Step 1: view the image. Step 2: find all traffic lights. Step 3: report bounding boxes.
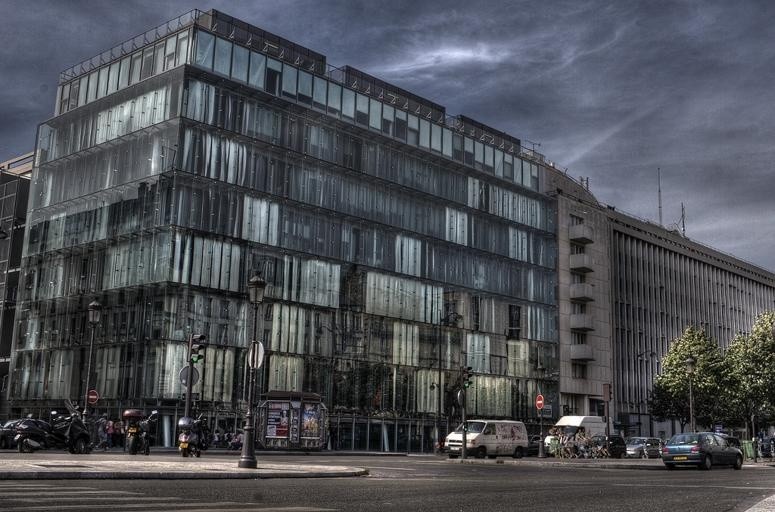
[460,366,473,389]
[190,334,207,362]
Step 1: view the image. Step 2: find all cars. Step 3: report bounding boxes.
[626,437,663,458]
[662,432,744,469]
[593,434,627,460]
[529,434,540,450]
[2,418,68,446]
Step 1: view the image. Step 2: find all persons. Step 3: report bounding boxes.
[281,408,317,443]
[545,426,611,460]
[87,412,128,452]
[216,425,244,451]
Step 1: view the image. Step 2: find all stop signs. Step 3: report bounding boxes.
[88,389,99,404]
[536,395,544,410]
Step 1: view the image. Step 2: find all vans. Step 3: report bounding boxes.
[444,420,528,458]
[544,415,605,454]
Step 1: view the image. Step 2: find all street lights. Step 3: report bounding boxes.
[83,301,102,438]
[685,355,695,434]
[536,363,547,458]
[238,277,267,467]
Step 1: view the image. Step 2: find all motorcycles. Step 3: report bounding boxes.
[123,409,156,455]
[177,413,203,458]
[16,418,49,453]
[65,413,93,453]
